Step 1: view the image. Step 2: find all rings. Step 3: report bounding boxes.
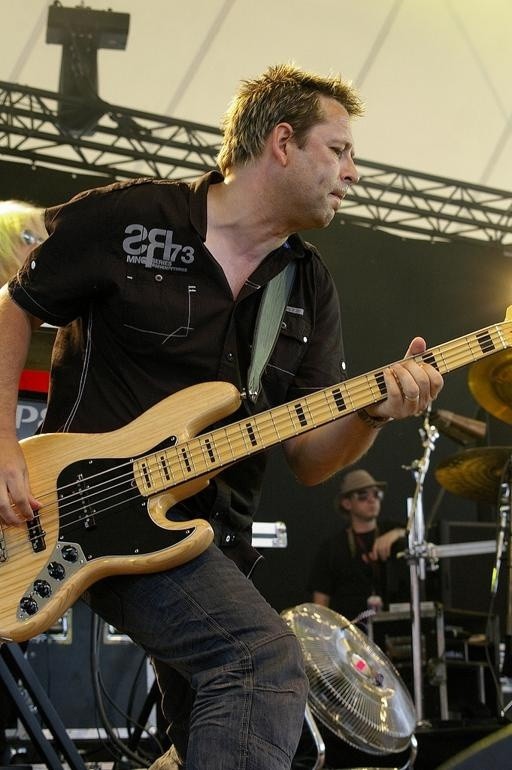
[404,396,419,402]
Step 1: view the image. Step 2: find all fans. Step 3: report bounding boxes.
[279,606,422,768]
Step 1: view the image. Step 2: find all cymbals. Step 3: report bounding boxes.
[468,346,511,424]
[436,447,511,507]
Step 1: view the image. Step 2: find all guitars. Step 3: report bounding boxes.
[1,319,512,643]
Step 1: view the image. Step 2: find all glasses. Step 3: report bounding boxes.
[18,228,45,248]
[353,490,385,501]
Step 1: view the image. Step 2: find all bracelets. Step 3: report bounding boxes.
[355,410,394,430]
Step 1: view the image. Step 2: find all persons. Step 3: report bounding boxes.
[0,66,445,770]
[0,200,49,288]
[309,470,409,652]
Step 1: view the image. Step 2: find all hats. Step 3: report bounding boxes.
[337,469,389,495]
